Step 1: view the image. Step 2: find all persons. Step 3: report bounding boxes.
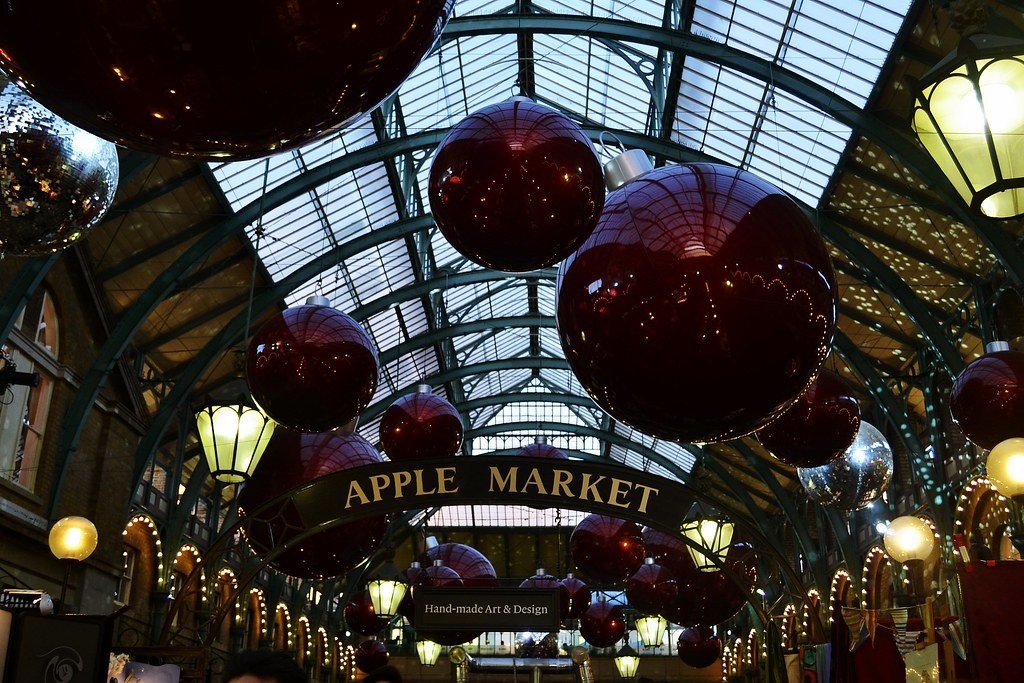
[219,648,309,683]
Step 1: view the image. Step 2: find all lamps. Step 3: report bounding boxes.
[634,612,668,650]
[188,155,278,485]
[614,594,642,681]
[905,0,1024,219]
[0,517,130,623]
[366,324,409,621]
[415,508,443,668]
[883,515,934,605]
[678,446,735,575]
[987,437,1024,560]
